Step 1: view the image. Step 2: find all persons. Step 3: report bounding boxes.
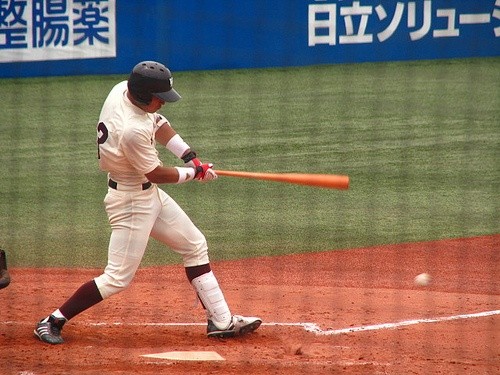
[33,60,262,344]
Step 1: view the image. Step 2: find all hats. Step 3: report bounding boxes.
[128,60,182,105]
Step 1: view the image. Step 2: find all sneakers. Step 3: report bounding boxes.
[33,317,65,345]
[207,314,262,339]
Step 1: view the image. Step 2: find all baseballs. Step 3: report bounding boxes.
[413,273,431,287]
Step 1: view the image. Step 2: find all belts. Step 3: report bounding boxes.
[108,178,152,191]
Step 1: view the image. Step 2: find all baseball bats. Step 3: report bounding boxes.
[213,170,350,189]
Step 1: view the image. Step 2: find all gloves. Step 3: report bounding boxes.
[181,151,203,168]
[192,163,218,184]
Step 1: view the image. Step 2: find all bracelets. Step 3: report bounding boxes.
[166,134,190,159]
[175,166,195,184]
